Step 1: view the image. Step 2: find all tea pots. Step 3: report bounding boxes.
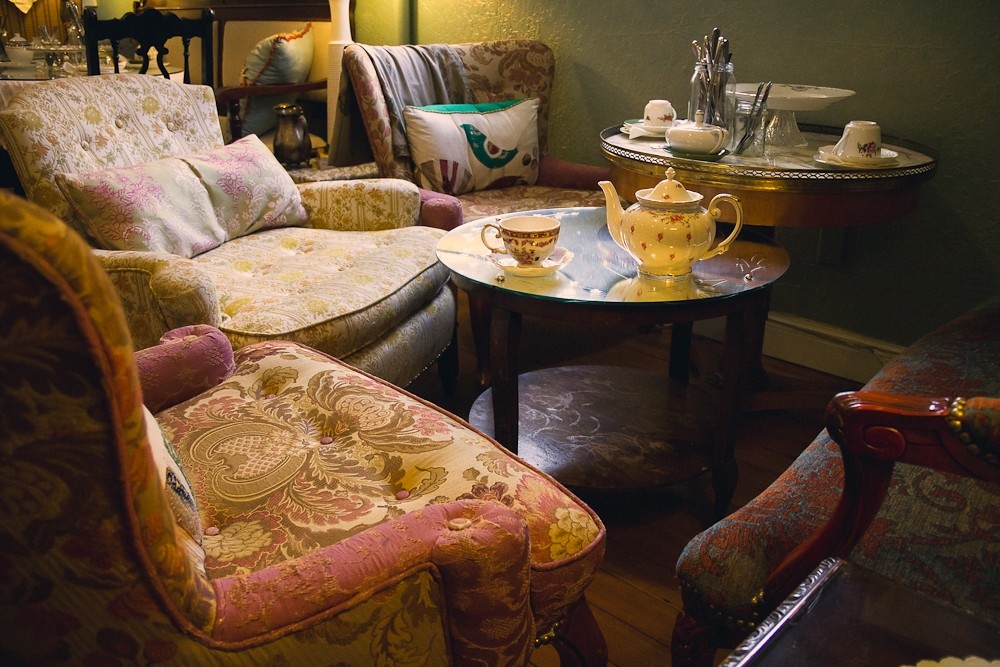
[597,167,744,278]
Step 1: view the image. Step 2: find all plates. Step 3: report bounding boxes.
[76,67,126,73]
[486,246,574,277]
[130,63,170,69]
[812,144,900,168]
[0,62,37,67]
[621,117,666,139]
[724,81,857,111]
[667,148,730,162]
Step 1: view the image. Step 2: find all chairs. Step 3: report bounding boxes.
[214,9,332,171]
[84,8,214,87]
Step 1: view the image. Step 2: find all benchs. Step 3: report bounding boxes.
[668,296,1000,667]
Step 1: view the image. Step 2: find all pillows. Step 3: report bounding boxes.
[402,98,540,196]
[239,21,314,137]
[53,134,307,258]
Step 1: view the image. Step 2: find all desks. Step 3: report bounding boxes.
[0,59,185,84]
[720,555,1000,666]
[597,121,942,414]
[433,207,790,529]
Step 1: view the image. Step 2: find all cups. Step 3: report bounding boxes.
[481,215,560,266]
[643,99,676,127]
[837,121,882,158]
[664,109,730,154]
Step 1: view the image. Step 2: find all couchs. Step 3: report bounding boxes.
[343,40,612,223]
[0,190,609,667]
[0,73,462,404]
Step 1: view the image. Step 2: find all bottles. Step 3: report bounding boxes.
[689,61,736,149]
[736,97,767,158]
